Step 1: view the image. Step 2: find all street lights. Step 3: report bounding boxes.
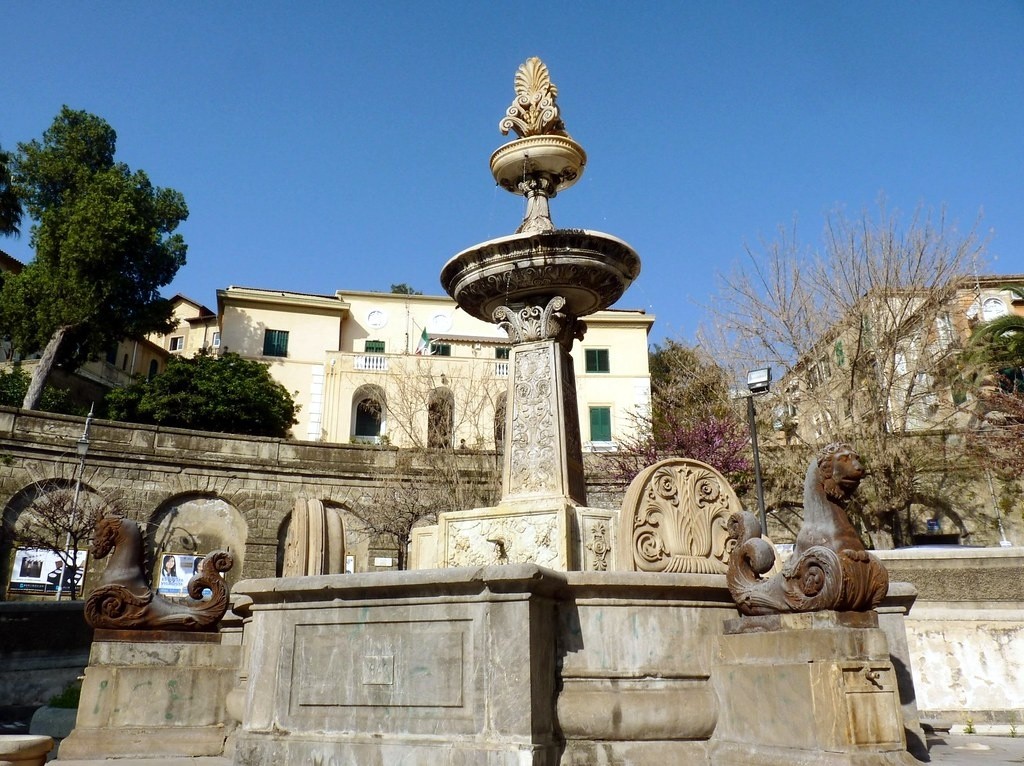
[733,369,771,543]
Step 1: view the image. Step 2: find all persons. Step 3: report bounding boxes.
[193,557,205,576]
[162,556,177,577]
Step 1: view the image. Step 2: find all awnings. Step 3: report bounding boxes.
[425,333,512,344]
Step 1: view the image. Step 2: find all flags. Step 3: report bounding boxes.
[415,326,429,354]
